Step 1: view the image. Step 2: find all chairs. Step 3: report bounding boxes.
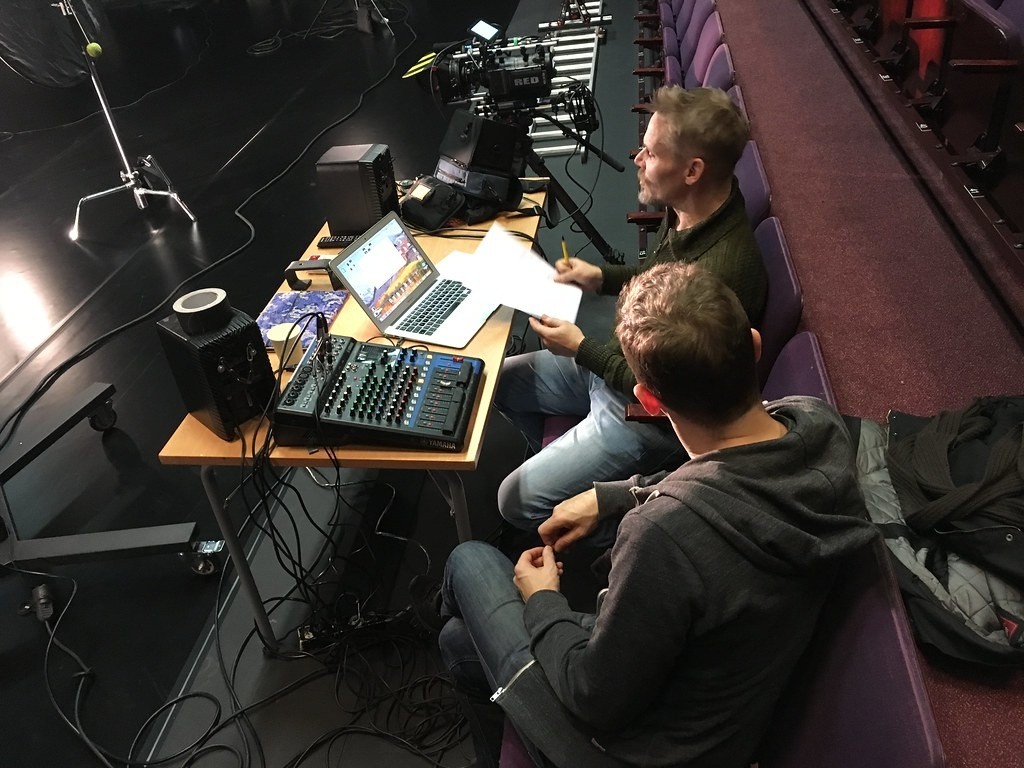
[630,0,949,768]
[907,0,1024,167]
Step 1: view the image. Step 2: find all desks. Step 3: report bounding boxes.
[157,178,549,654]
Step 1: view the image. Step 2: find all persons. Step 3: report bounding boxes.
[406,261,881,768]
[491,86,769,533]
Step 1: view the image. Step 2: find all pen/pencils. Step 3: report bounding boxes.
[561,234,572,269]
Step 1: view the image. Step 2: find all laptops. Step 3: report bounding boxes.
[328,210,502,349]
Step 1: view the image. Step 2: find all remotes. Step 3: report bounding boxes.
[319,235,363,248]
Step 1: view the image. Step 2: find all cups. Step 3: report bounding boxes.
[267,322,303,368]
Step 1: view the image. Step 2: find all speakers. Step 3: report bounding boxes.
[316,143,401,237]
[156,302,280,443]
[438,108,520,177]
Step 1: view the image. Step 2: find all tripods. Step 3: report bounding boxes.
[303,1,395,41]
[51,0,198,236]
